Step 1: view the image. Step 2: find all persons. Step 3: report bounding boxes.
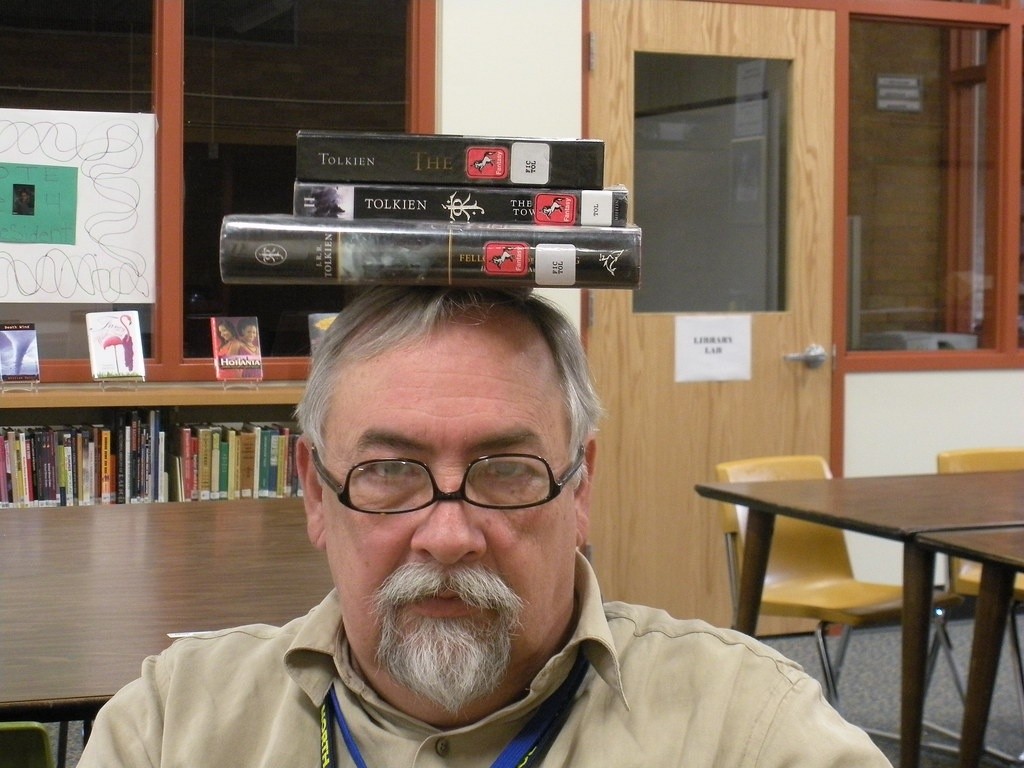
[217,320,260,377]
[78,285,896,768]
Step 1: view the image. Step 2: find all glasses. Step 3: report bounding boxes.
[310,441,585,515]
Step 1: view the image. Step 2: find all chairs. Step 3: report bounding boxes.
[0,721,68,768]
[923,446,1024,768]
[712,456,969,756]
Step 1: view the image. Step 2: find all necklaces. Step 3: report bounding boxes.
[247,343,255,347]
[244,341,257,352]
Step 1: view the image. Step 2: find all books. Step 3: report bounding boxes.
[0,323,40,380]
[309,314,340,356]
[219,131,643,290]
[0,410,305,509]
[86,311,146,381]
[210,317,263,381]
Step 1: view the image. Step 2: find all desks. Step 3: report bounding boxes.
[0,383,307,407]
[0,497,335,722]
[693,468,1024,768]
